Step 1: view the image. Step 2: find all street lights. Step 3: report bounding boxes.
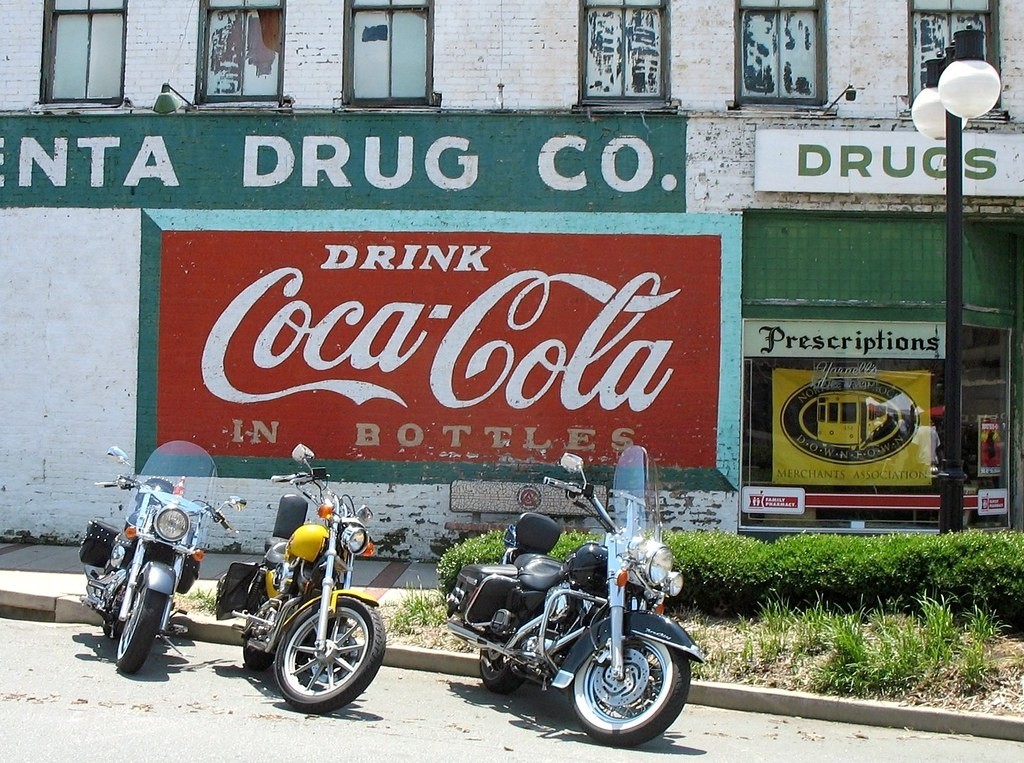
[911,22,1002,535]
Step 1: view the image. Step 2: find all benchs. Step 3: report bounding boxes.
[444,479,608,541]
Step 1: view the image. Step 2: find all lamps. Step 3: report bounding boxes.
[822,83,857,117]
[152,83,194,114]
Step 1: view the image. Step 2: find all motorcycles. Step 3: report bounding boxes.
[213,443,387,715]
[77,439,247,674]
[442,443,706,750]
